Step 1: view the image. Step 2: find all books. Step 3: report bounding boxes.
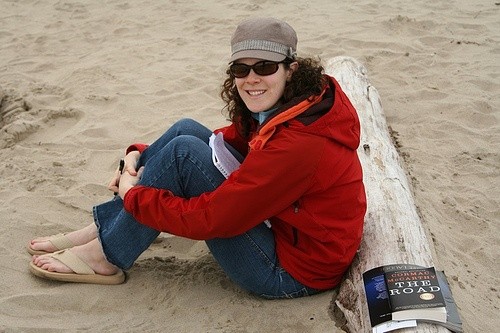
[362,264,463,333]
[209,132,271,228]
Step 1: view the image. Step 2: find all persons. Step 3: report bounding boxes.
[25,17,366,299]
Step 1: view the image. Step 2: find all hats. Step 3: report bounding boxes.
[228,17,298,65]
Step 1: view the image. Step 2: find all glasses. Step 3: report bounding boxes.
[230,59,291,78]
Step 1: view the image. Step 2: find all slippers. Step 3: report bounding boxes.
[27,232,77,257]
[28,246,124,285]
